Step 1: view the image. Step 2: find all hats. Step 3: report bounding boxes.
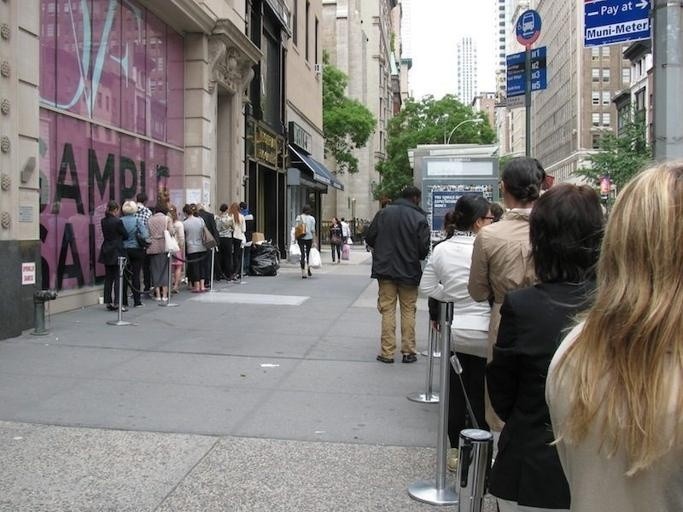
[301,204,313,213]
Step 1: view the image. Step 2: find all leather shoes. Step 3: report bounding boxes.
[106,287,208,312]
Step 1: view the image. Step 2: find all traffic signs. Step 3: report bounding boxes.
[504,44,547,97]
[515,9,543,47]
[583,0,652,48]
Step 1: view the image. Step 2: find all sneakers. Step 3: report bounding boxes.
[403,354,417,363]
[377,355,394,363]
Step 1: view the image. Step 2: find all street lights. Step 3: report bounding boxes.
[444,114,484,142]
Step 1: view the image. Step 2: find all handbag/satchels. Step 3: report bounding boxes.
[308,242,321,269]
[199,216,217,249]
[163,216,181,253]
[134,219,152,247]
[295,215,306,240]
[347,236,353,244]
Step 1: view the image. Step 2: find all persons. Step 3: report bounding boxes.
[362,220,371,252]
[295,204,317,279]
[365,187,430,364]
[416,157,683,511]
[96,190,254,312]
[329,217,344,264]
[340,217,351,242]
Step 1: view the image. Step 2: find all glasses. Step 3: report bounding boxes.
[473,213,495,223]
